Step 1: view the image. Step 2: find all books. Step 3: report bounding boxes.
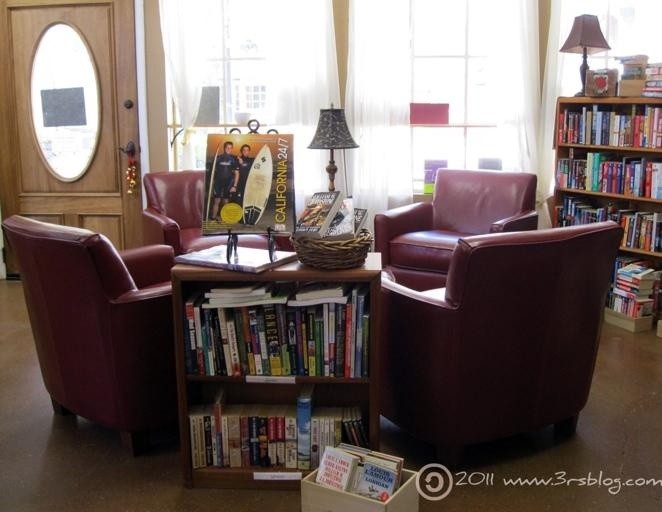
[294,192,368,243]
[554,56,662,327]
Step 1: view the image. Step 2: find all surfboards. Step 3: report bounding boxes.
[242,144,273,226]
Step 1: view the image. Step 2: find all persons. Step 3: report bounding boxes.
[230,144,255,204]
[210,141,239,223]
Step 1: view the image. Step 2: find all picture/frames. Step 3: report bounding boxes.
[585,68,618,97]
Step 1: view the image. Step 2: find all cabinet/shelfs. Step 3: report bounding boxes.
[550,97,662,339]
[169,251,382,487]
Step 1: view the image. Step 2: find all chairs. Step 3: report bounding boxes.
[362,217,622,474]
[1,214,197,459]
[372,169,542,291]
[142,171,278,265]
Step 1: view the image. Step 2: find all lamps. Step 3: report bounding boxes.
[559,13,611,97]
[306,103,358,191]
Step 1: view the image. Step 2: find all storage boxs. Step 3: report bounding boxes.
[299,463,420,512]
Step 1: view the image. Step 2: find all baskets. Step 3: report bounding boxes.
[289,229,375,271]
